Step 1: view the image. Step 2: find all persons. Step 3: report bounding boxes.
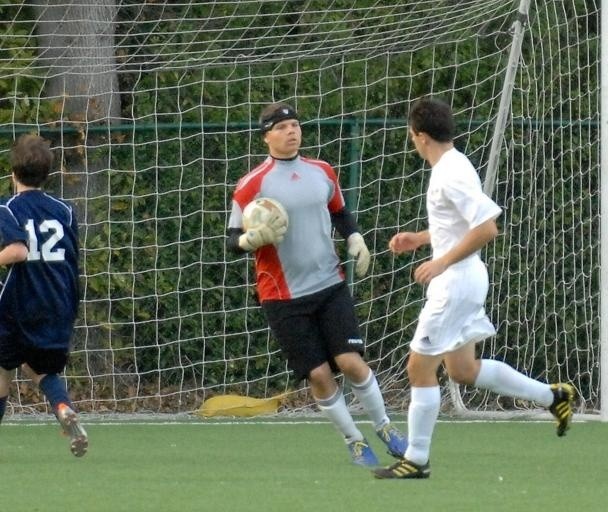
[224,99,409,468]
[371,95,575,480]
[0,129,88,457]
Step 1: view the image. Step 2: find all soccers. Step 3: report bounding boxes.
[241,197,290,236]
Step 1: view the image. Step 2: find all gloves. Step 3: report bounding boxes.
[238,208,287,253]
[346,231,371,278]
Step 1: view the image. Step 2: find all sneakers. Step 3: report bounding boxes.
[373,416,409,460]
[344,431,380,468]
[544,382,575,438]
[58,403,89,458]
[371,457,431,480]
